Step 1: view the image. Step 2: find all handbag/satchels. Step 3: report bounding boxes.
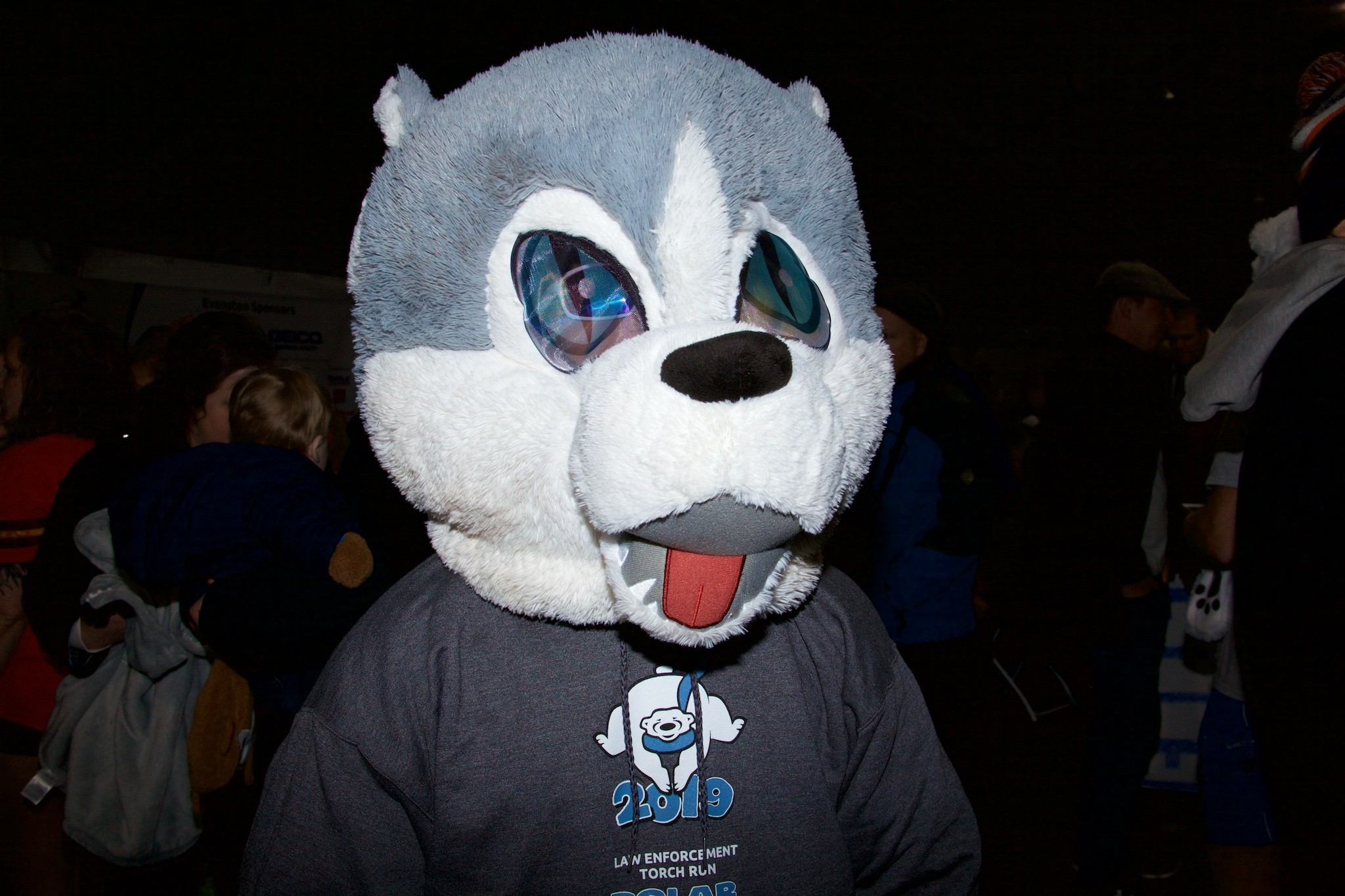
[188,656,260,816]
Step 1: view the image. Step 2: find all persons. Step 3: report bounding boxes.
[1,307,434,896]
[832,285,976,642]
[1019,48,1345,896]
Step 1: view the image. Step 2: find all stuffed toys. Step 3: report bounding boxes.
[236,28,986,896]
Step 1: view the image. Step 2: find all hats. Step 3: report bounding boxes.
[1291,52,1345,149]
[1100,263,1192,302]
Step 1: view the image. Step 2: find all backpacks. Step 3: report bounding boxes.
[876,384,1015,555]
